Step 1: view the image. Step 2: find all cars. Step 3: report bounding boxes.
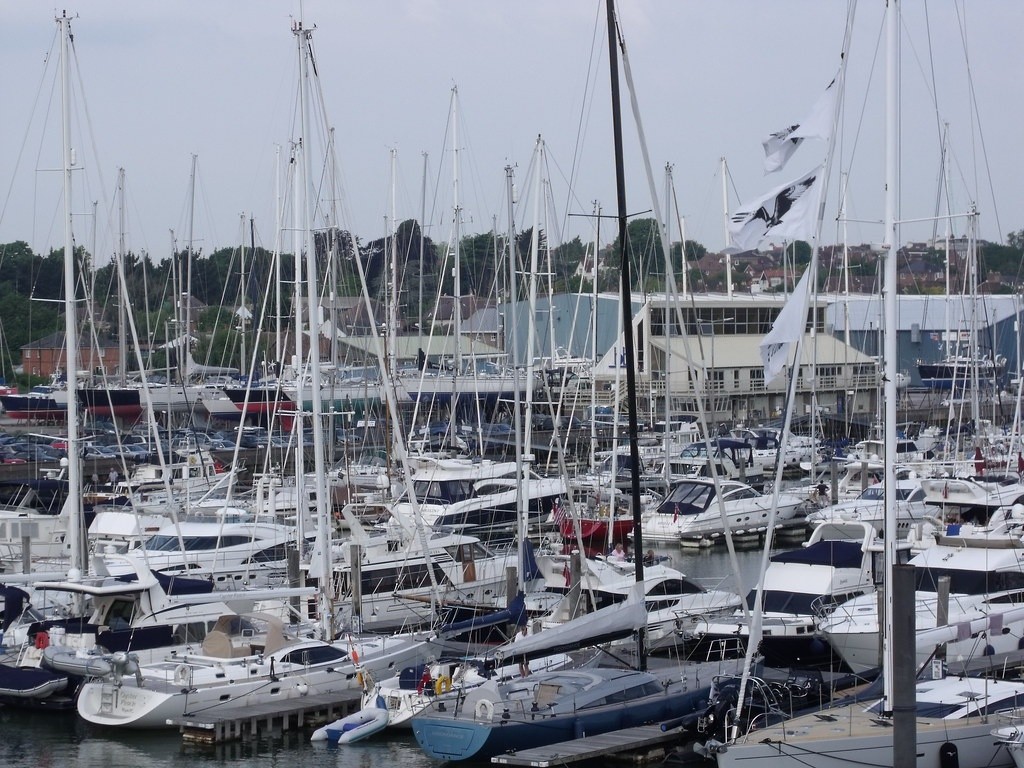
[261,436,285,447]
[482,423,516,437]
[223,432,269,447]
[520,414,548,431]
[559,414,592,429]
[277,435,310,447]
[80,420,123,435]
[80,446,117,461]
[103,443,152,462]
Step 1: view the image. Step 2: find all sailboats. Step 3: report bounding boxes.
[0,2,1024,768]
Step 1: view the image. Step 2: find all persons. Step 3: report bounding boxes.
[612,543,625,558]
[812,480,831,508]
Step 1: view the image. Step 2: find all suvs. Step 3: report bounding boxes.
[589,413,629,429]
[311,428,362,447]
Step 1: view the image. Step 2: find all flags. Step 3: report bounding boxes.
[758,262,811,388]
[762,68,839,176]
[673,507,679,523]
[562,566,571,586]
[729,159,824,251]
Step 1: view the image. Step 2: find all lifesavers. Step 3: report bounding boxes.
[434,674,451,696]
[475,698,495,721]
[34,632,49,649]
[173,664,190,683]
